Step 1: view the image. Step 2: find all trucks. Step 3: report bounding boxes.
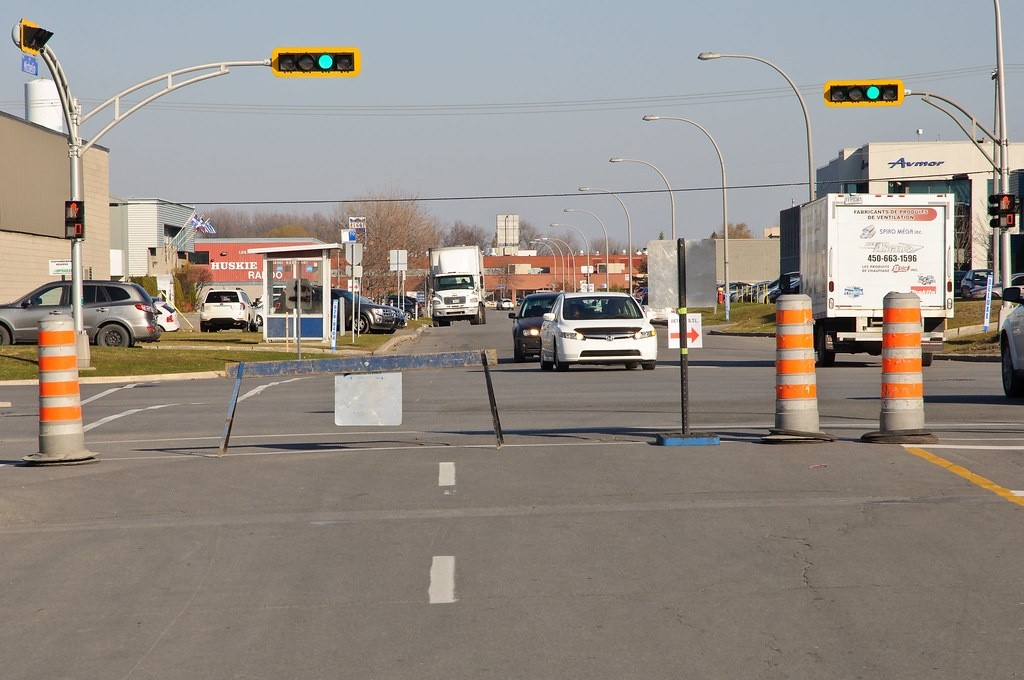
[799,193,955,367]
[428,244,486,327]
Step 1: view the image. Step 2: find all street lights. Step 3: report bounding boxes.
[578,186,634,297]
[528,237,577,293]
[609,157,676,240]
[563,208,609,292]
[641,114,731,323]
[550,223,590,293]
[698,51,815,202]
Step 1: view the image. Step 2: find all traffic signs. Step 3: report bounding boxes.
[668,313,702,350]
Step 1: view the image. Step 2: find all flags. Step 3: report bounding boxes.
[189,214,216,236]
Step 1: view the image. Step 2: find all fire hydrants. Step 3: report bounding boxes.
[718,287,724,304]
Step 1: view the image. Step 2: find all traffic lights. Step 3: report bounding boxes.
[21,17,54,58]
[823,79,905,107]
[65,200,85,239]
[270,46,361,78]
[998,195,1017,228]
[988,194,1001,228]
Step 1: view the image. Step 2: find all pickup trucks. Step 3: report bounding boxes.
[497,298,514,310]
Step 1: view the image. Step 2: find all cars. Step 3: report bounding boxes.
[331,288,407,336]
[999,287,1024,401]
[717,270,800,304]
[153,300,180,333]
[517,299,524,306]
[508,292,597,363]
[254,285,285,327]
[538,291,657,372]
[954,268,1024,302]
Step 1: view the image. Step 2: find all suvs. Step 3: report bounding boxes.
[0,279,161,348]
[373,294,424,321]
[199,287,257,333]
